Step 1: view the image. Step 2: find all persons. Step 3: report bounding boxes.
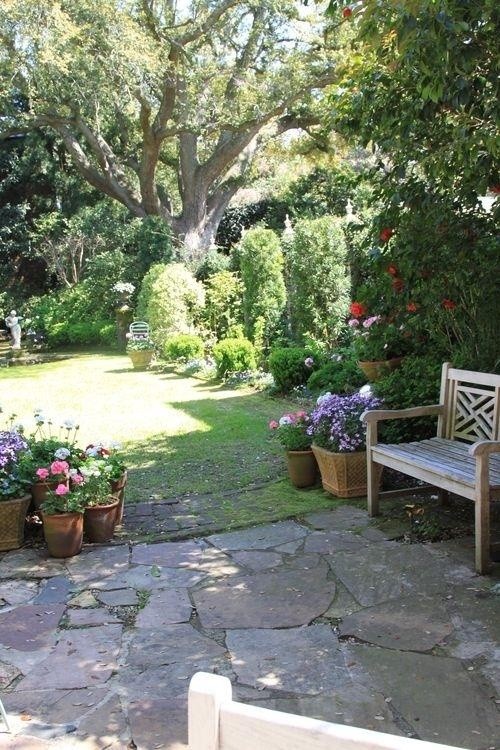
[5,310,23,349]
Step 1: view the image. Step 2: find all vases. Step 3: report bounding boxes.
[310,444,384,499]
[286,451,317,489]
[127,350,155,369]
[0,469,127,558]
[119,291,129,303]
[355,356,406,383]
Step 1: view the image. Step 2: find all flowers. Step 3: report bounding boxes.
[348,314,405,362]
[125,333,154,352]
[0,405,130,514]
[111,282,136,293]
[266,392,384,452]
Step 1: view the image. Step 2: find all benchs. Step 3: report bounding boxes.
[359,361,500,576]
